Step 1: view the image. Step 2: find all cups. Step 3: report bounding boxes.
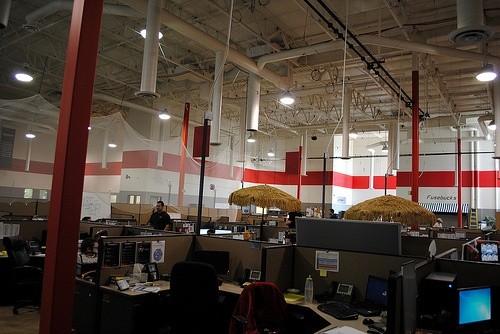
[244,232,250,240]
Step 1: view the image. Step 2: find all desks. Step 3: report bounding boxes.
[0,218,500,334]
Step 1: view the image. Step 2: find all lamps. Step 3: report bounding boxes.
[14,63,34,82]
[159,108,170,119]
[247,132,256,142]
[488,120,496,130]
[125,174,131,178]
[267,146,275,156]
[26,129,36,138]
[279,88,295,104]
[141,25,163,41]
[476,39,496,81]
[382,130,388,152]
[108,138,117,147]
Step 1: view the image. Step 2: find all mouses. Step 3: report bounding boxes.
[362,318,374,325]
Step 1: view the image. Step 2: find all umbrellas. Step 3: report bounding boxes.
[343,194,438,226]
[228,184,302,239]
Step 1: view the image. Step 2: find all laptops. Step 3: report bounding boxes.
[354,275,389,316]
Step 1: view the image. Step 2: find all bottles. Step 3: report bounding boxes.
[305,275,313,303]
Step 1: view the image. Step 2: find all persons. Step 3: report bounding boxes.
[76,239,97,264]
[435,218,443,227]
[285,211,300,229]
[147,201,172,231]
[330,209,338,219]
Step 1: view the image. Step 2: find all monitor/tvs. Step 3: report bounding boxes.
[454,286,496,327]
[192,250,230,276]
[200,229,233,235]
[478,243,499,262]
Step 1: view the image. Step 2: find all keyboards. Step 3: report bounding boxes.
[318,303,359,320]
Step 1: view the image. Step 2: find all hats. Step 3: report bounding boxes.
[437,218,443,222]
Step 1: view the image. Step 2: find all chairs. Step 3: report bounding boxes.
[4,235,44,315]
[229,283,289,334]
[163,262,224,334]
